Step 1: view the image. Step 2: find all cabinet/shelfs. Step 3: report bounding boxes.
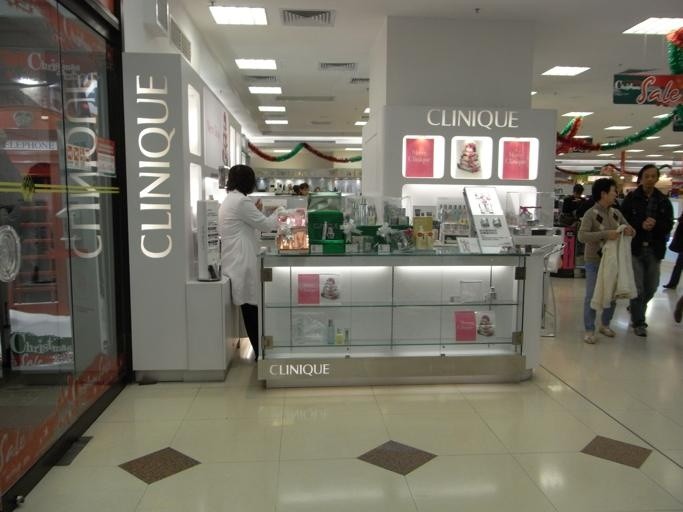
[128,46,250,385]
[259,249,550,390]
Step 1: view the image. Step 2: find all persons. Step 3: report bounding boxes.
[292,184,321,197]
[216,164,290,362]
[562,164,682,344]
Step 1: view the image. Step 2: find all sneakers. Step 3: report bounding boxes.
[634,323,647,337]
[663,284,677,290]
[599,326,616,337]
[584,328,597,345]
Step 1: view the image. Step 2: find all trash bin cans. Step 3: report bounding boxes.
[549,227,575,277]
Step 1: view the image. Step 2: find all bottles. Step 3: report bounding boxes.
[327,317,344,345]
